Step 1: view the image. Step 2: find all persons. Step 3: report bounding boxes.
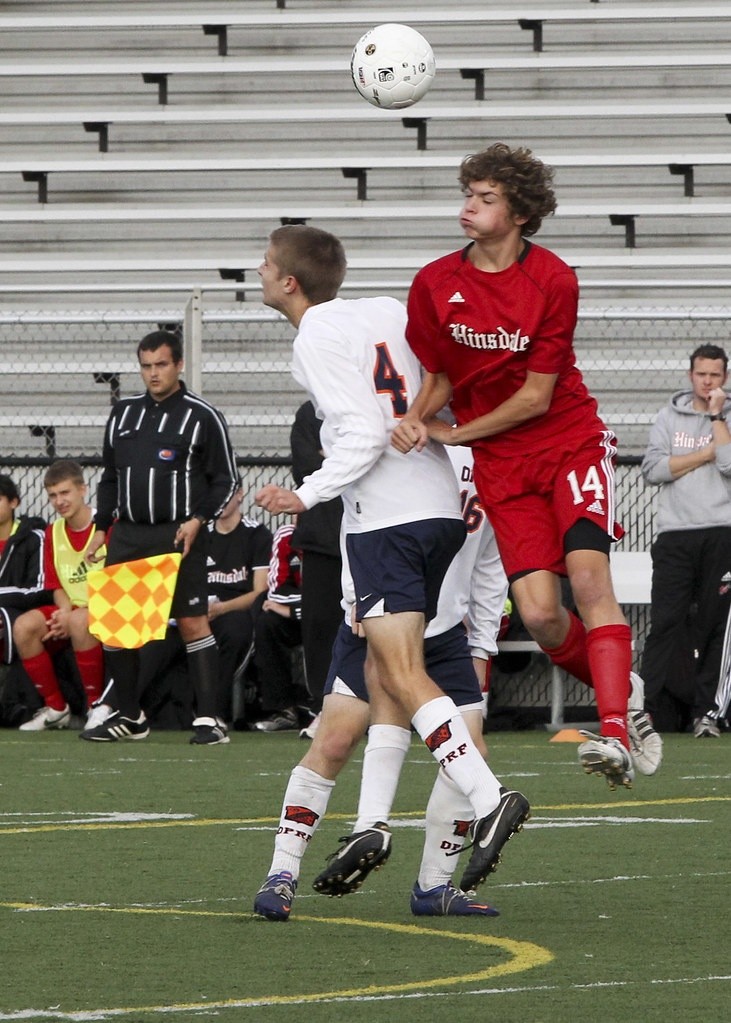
[79,331,237,745]
[389,145,663,791]
[291,399,368,739]
[637,344,731,737]
[0,474,53,728]
[255,433,511,921]
[138,471,273,733]
[13,460,117,730]
[254,513,312,732]
[461,585,513,732]
[252,225,532,899]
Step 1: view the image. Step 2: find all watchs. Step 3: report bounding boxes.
[710,412,727,422]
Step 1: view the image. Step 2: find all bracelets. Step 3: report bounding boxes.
[194,515,207,527]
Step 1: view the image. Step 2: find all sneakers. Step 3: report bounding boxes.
[577,730,634,790]
[252,706,298,731]
[254,871,297,920]
[410,879,500,916]
[445,787,531,892]
[80,709,150,742]
[299,712,321,739]
[312,821,392,900]
[83,702,114,730]
[190,715,230,745]
[692,715,719,737]
[19,704,70,731]
[623,671,663,777]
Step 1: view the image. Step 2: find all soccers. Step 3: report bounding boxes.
[347,23,439,111]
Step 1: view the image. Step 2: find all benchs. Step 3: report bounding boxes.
[496,549,653,730]
[0,0,730,456]
